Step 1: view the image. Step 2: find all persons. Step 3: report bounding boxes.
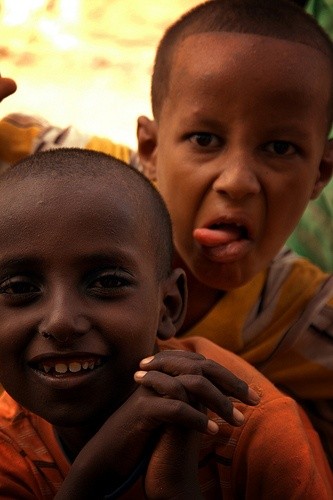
[1,0,332,446]
[0,148,333,499]
